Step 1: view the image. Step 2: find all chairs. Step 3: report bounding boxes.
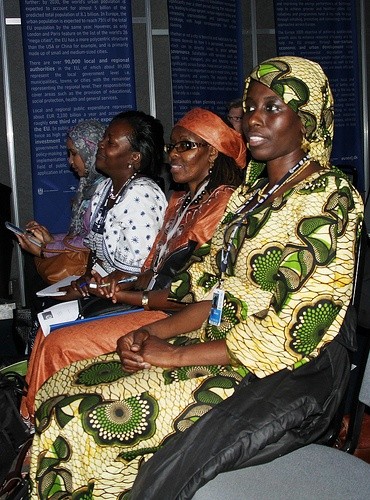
[192,349,370,500]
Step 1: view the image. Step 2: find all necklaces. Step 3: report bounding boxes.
[179,185,210,214]
[256,162,310,203]
[103,173,138,208]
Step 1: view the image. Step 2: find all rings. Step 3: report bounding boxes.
[18,243,21,245]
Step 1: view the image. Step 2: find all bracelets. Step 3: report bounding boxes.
[141,290,150,311]
[39,249,44,260]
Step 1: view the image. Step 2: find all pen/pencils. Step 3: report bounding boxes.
[78,282,89,288]
[98,275,138,288]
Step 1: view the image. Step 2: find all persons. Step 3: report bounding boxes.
[0,55,365,500]
[20,108,247,432]
[4,109,173,357]
[226,100,247,136]
[17,120,111,260]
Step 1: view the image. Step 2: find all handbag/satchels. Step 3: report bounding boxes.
[34,234,91,286]
[0,371,31,430]
[0,473,35,500]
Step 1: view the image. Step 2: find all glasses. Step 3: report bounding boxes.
[227,116,242,122]
[163,140,210,154]
